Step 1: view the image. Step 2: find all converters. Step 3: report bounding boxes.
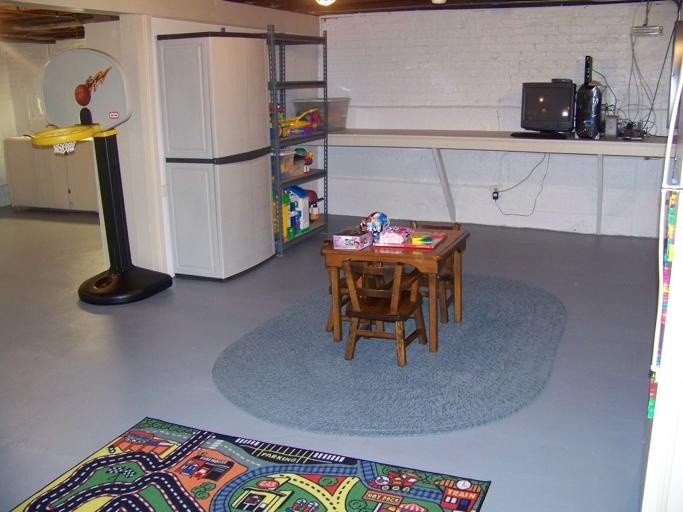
[493,192,498,199]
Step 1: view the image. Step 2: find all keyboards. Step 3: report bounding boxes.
[510,132,567,140]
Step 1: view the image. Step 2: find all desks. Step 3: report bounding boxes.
[321,228,469,353]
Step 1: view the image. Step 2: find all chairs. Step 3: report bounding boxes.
[325,231,390,334]
[393,221,460,324]
[344,265,427,366]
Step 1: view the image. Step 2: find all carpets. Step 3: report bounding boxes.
[212,273,568,438]
[10,416,492,512]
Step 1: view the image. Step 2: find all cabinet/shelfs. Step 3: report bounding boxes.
[267,23,328,257]
[3,135,99,215]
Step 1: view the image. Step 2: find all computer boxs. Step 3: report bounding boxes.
[574,82,607,139]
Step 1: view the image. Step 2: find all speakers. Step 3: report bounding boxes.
[606,117,616,138]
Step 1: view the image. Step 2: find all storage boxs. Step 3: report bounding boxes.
[293,97,350,131]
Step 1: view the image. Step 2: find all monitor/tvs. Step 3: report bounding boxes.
[520,83,576,132]
